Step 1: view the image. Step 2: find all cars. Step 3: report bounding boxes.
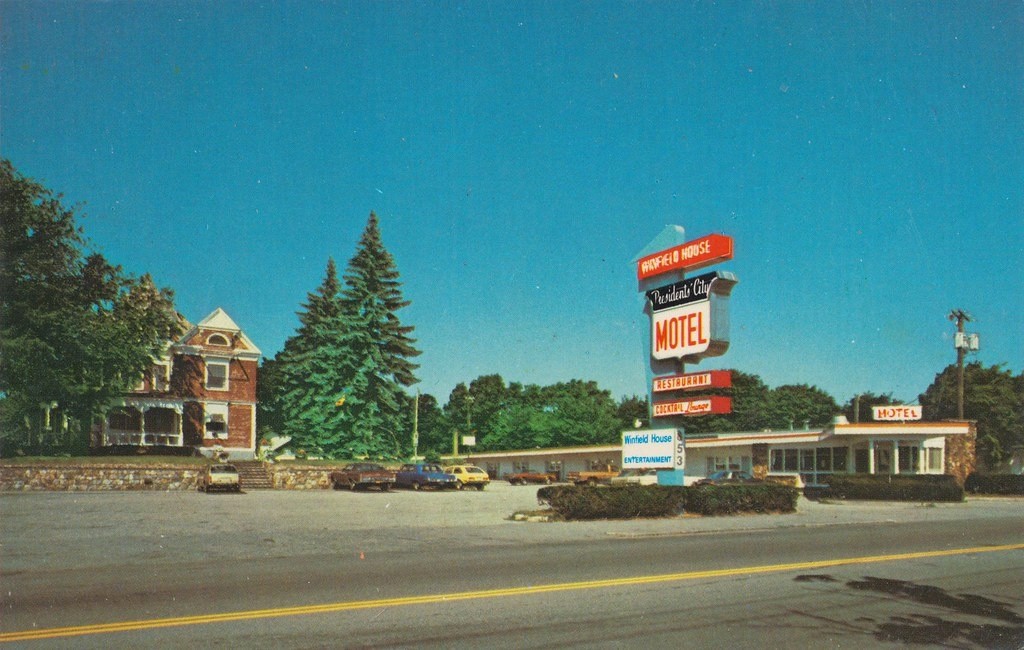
[445,466,490,490]
[398,464,456,489]
[506,469,557,485]
[197,464,240,492]
[330,463,397,491]
[707,470,752,484]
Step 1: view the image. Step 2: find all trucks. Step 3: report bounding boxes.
[566,463,621,487]
